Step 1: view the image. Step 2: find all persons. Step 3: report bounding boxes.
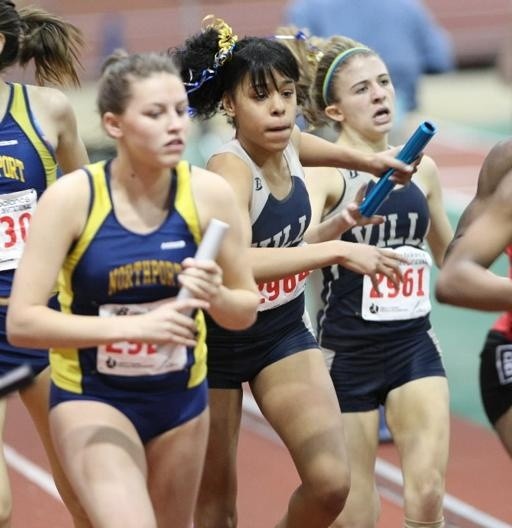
[174,14,422,527]
[276,28,450,528]
[443,143,512,273]
[6,50,260,528]
[0,2,94,528]
[433,176,512,468]
